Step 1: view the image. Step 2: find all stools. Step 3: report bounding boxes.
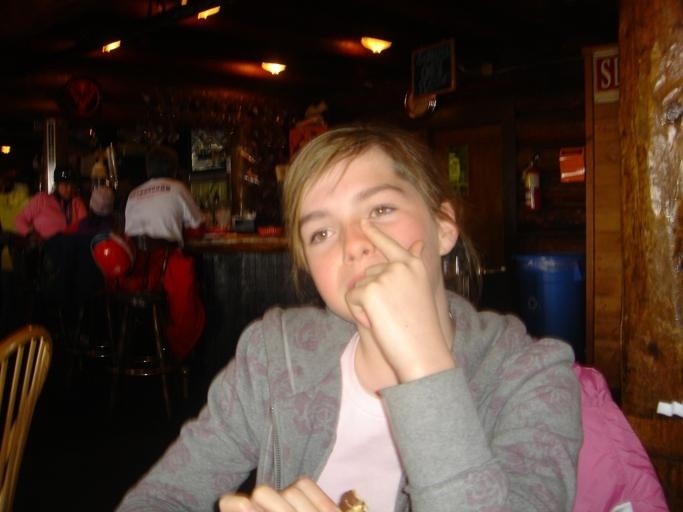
[108,289,172,422]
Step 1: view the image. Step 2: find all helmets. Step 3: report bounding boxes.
[53,167,76,182]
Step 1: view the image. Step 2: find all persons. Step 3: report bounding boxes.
[114,126,581,512]
[572,359,666,507]
[125,147,202,248]
[77,184,115,234]
[14,166,87,241]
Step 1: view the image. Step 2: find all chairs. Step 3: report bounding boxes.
[0,324,53,511]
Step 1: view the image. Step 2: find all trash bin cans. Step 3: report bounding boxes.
[509,251,585,337]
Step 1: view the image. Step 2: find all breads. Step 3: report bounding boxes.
[339,491,369,512]
